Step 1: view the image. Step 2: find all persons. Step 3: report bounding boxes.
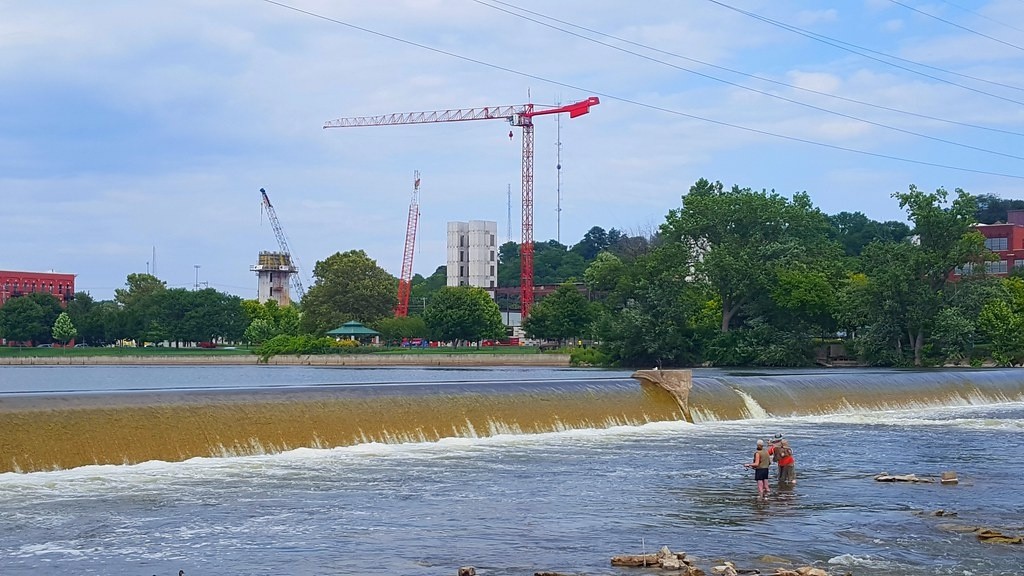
[768,433,794,481]
[744,439,771,492]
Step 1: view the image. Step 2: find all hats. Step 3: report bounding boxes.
[774,433,782,440]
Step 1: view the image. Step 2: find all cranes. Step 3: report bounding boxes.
[393,169,423,317]
[323,96,601,334]
[259,188,309,304]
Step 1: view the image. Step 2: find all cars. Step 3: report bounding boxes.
[196,341,216,349]
[37,343,52,348]
[75,342,88,347]
